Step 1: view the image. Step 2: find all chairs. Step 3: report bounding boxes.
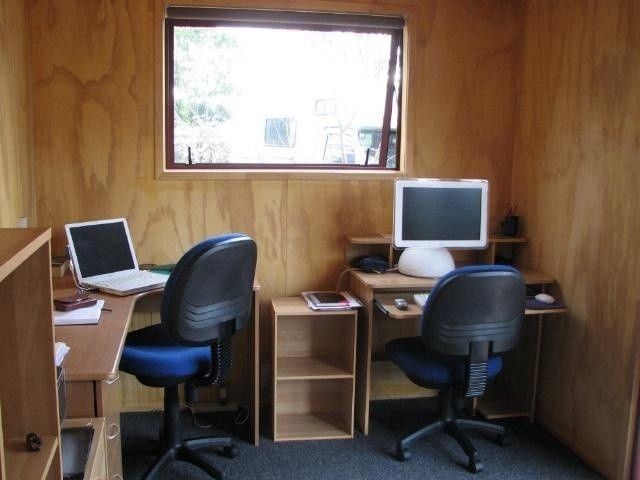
[384,264,528,472]
[118,233,258,479]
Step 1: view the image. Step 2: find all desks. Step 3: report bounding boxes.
[344,234,569,437]
[51,264,262,479]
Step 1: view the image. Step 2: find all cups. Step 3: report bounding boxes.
[503,215,518,237]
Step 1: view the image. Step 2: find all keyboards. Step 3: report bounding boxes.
[412,291,431,309]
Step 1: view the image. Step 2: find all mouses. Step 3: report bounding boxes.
[534,292,556,304]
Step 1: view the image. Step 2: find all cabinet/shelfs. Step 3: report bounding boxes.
[268,296,360,442]
[0,226,63,480]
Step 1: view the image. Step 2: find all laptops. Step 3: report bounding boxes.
[64,216,169,298]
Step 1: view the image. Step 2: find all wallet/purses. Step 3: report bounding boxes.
[53,295,98,312]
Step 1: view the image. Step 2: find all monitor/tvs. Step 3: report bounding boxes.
[393,177,489,277]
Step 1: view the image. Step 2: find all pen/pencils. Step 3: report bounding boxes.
[508,205,517,216]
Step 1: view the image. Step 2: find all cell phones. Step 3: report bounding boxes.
[393,297,410,309]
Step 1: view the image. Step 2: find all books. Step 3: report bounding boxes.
[53,299,105,325]
[301,291,364,310]
